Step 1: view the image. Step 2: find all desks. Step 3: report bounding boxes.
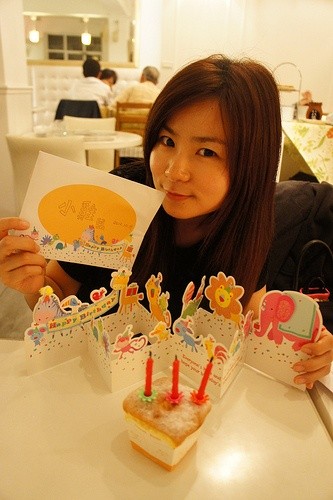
[0,339,333,500]
[26,129,143,167]
[274,118,333,185]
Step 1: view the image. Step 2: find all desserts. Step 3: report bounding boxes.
[122,377,212,471]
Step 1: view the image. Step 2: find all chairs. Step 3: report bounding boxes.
[55,100,102,119]
[62,115,117,173]
[115,101,152,168]
[6,135,85,215]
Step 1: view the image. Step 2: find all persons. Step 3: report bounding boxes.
[64,57,115,110]
[0,52,333,390]
[98,68,117,89]
[118,63,162,111]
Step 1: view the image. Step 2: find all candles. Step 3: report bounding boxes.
[171,354,179,399]
[145,351,153,396]
[197,356,214,400]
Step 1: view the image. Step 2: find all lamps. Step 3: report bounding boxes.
[29,17,40,42]
[81,17,91,46]
